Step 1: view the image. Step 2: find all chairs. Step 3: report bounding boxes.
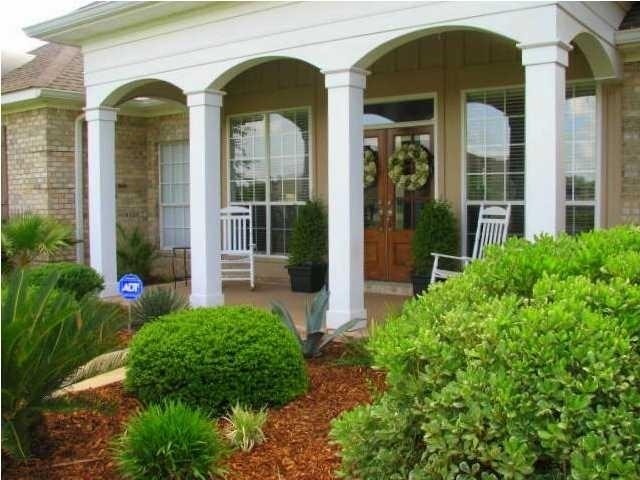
[219,201,258,290]
[429,202,512,287]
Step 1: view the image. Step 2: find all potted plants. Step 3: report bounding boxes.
[410,198,460,300]
[284,198,329,294]
